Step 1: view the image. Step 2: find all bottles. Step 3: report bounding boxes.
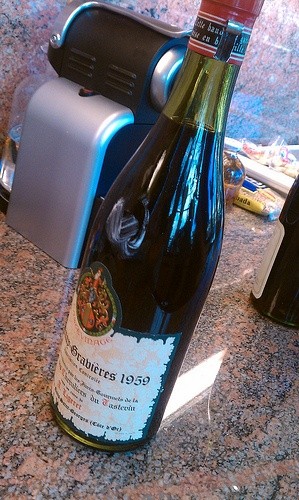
[219,145,245,215]
[46,1,270,453]
[249,158,298,329]
